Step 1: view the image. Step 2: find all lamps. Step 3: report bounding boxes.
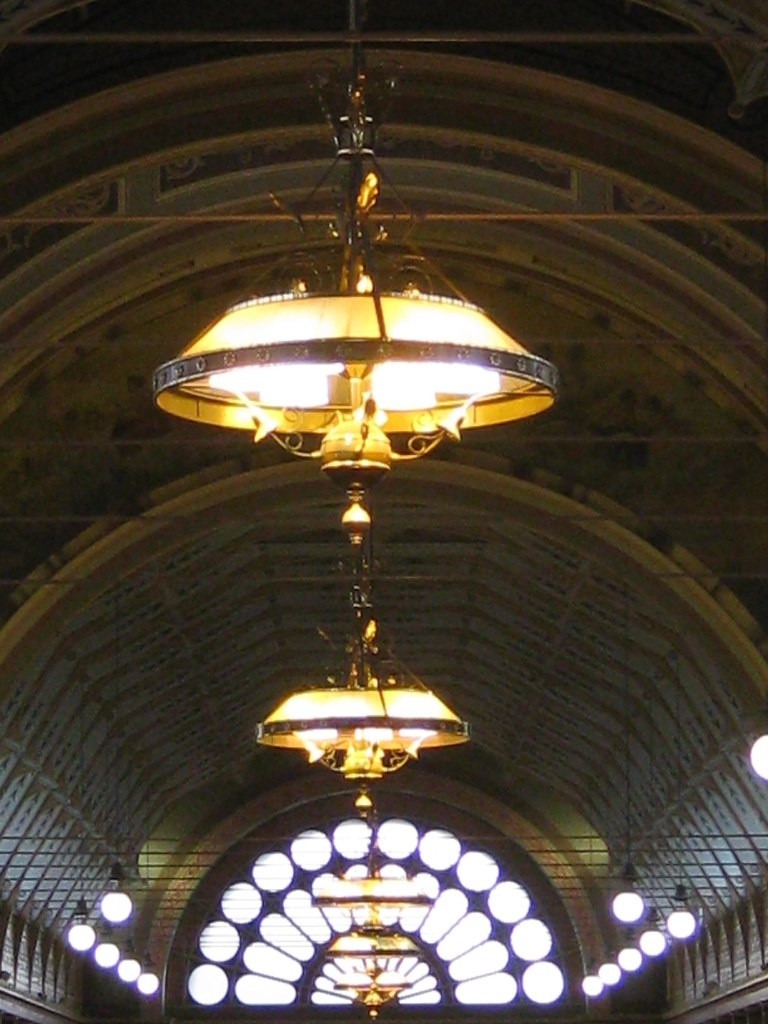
[66,593,160,995]
[583,585,695,997]
[313,792,437,1023]
[150,1,558,544]
[257,485,471,817]
